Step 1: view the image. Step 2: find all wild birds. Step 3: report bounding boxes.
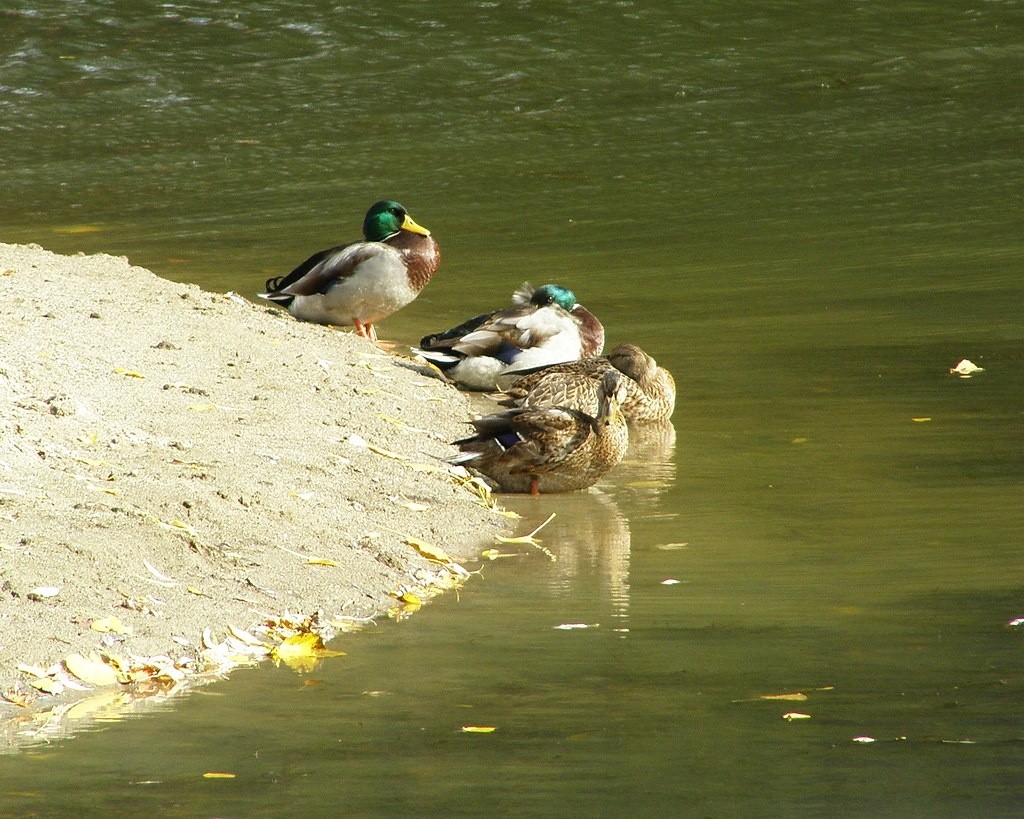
[489,341,678,428]
[448,371,629,497]
[497,485,634,641]
[602,419,674,504]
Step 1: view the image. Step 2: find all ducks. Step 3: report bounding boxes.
[422,283,604,389]
[257,200,441,341]
